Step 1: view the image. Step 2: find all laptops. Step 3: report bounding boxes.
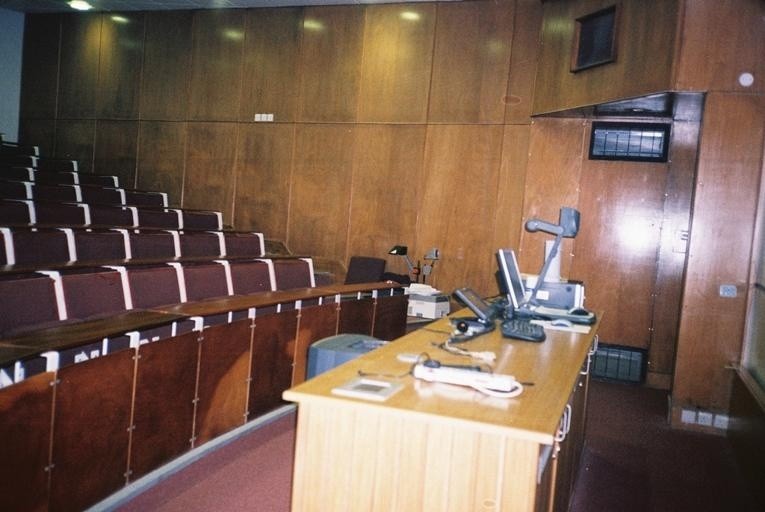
[494,249,596,325]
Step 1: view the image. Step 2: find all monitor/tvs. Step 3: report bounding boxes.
[449,287,496,332]
[344,256,387,285]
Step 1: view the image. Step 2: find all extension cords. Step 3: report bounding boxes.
[413,362,523,399]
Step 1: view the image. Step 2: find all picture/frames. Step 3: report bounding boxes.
[568,2,621,73]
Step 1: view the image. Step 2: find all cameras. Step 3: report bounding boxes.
[388,244,408,255]
[423,247,441,260]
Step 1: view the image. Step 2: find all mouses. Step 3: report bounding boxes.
[567,306,588,316]
[551,319,571,327]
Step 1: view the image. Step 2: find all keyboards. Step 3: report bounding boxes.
[500,319,545,343]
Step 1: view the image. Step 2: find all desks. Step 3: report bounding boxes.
[0,281,408,512]
[1,196,183,211]
[0,253,317,265]
[282,295,605,511]
[0,219,260,235]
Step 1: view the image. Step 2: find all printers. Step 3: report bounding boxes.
[403,282,450,320]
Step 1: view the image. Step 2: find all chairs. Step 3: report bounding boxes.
[168,262,233,304]
[110,189,172,211]
[60,229,128,267]
[255,256,320,291]
[32,155,78,171]
[0,229,76,267]
[108,226,179,264]
[100,263,185,311]
[70,185,127,208]
[167,208,225,232]
[119,206,182,233]
[1,172,35,179]
[74,174,120,187]
[1,180,33,197]
[76,207,135,231]
[166,229,222,260]
[23,167,72,184]
[32,205,88,232]
[21,181,84,201]
[260,239,298,261]
[215,258,276,297]
[344,255,387,297]
[214,229,264,264]
[1,269,65,331]
[1,200,32,228]
[37,268,128,317]
[0,142,40,156]
[1,157,33,169]
